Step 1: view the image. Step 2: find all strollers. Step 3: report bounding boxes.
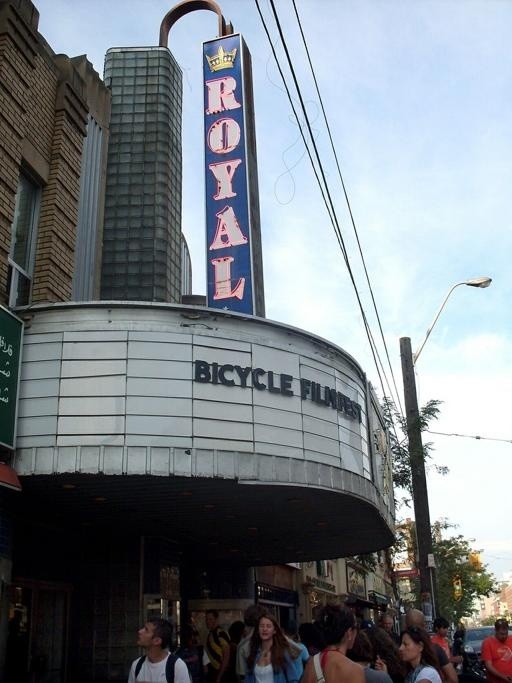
[456,650,488,683]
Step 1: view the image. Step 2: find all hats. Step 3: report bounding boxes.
[493,618,510,630]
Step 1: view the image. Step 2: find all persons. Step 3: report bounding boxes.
[175,600,512,683]
[126,616,192,681]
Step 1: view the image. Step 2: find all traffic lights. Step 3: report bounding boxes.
[452,578,463,603]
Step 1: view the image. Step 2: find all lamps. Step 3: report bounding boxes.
[301,578,314,594]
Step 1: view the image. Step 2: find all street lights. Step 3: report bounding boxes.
[399,275,496,627]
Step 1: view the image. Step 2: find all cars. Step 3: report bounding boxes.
[453,625,512,670]
